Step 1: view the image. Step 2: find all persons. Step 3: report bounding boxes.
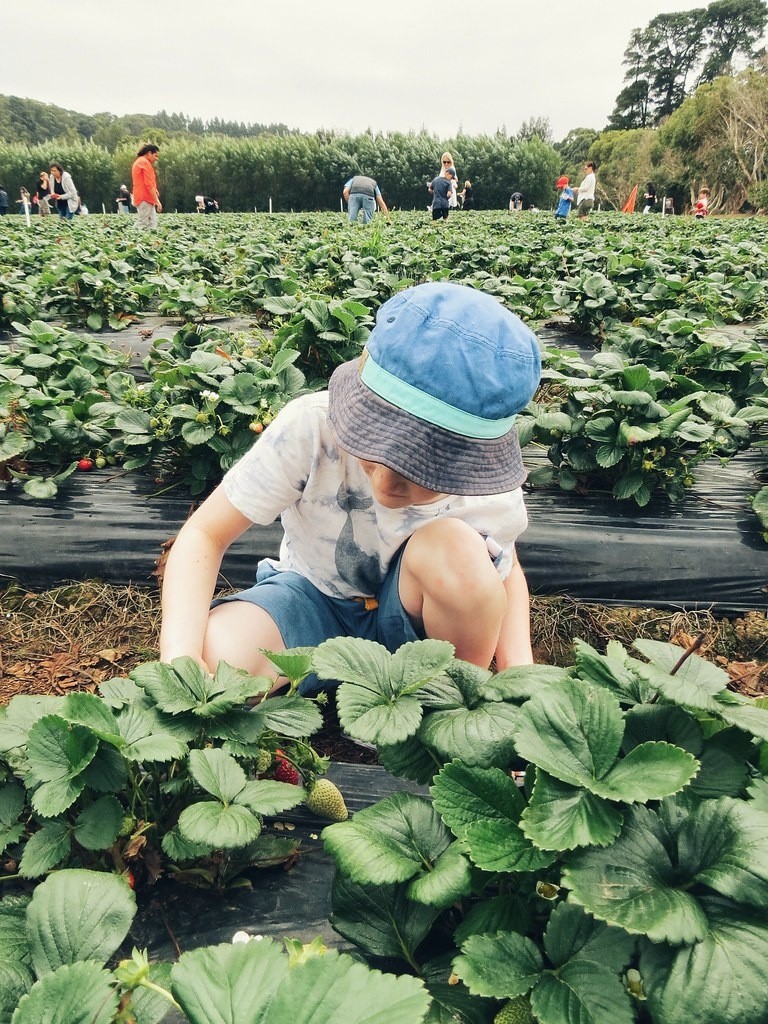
[554,177,574,219]
[509,193,539,211]
[195,195,219,214]
[161,282,542,707]
[343,176,395,225]
[33,162,88,220]
[0,185,8,216]
[643,181,655,214]
[428,152,473,220]
[689,188,710,219]
[572,161,596,220]
[15,187,32,214]
[132,144,162,228]
[116,185,130,215]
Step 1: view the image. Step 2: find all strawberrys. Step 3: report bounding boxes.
[228,312,284,359]
[494,989,541,1024]
[126,874,134,889]
[549,429,563,440]
[253,744,348,821]
[118,818,136,836]
[79,413,270,471]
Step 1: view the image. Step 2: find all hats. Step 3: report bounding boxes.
[556,177,569,188]
[446,168,456,180]
[325,281,542,496]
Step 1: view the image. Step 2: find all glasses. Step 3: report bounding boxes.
[443,160,451,164]
